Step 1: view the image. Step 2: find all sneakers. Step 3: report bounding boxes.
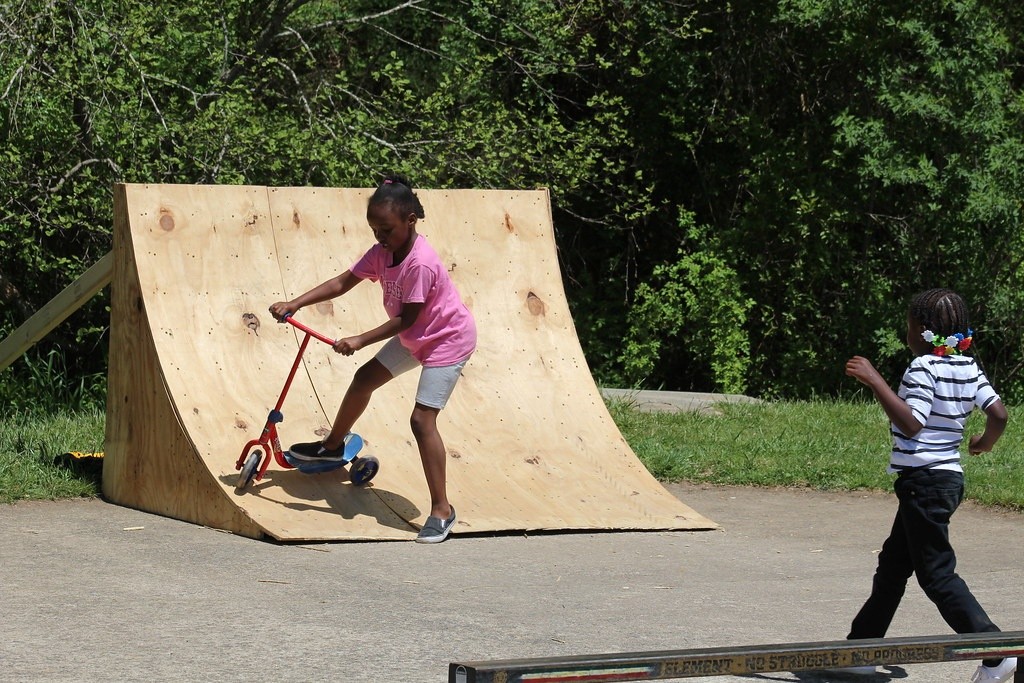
[971,656,1017,683]
[825,664,876,675]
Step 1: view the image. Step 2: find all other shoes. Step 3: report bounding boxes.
[416,504,456,543]
[288,441,344,461]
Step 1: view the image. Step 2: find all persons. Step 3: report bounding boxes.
[269,173,476,544]
[824,288,1017,683]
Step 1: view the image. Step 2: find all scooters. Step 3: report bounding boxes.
[233,306,381,489]
